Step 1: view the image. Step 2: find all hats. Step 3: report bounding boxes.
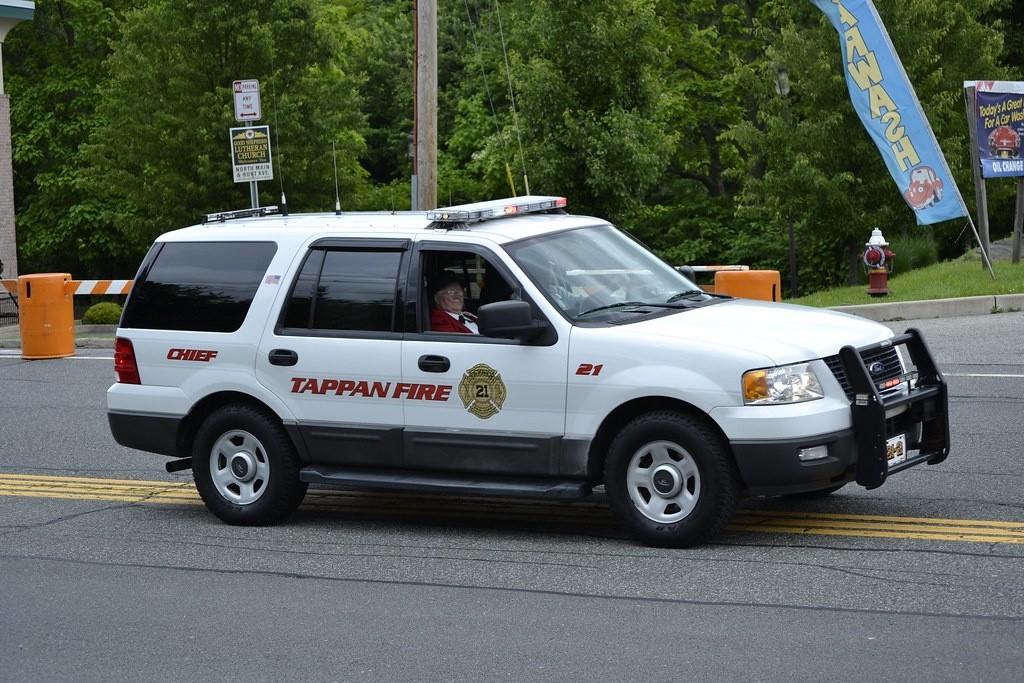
[424,270,466,297]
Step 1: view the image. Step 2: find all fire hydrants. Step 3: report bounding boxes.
[852,225,897,296]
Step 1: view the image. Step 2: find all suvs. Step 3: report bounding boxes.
[103,196,951,549]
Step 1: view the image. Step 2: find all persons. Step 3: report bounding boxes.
[512,251,629,317]
[430,271,479,334]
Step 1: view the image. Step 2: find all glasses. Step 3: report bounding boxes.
[439,289,463,296]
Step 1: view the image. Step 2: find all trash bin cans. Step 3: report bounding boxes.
[714,270,782,302]
[17,273,77,359]
